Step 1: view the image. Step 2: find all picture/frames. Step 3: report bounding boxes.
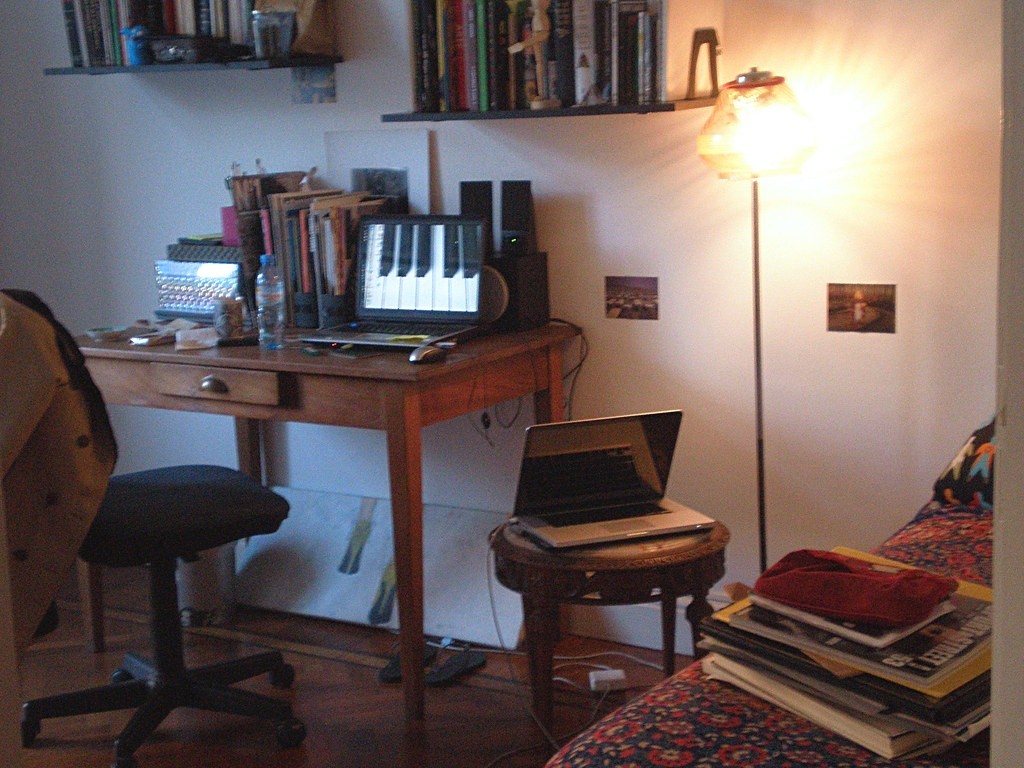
[323,128,431,216]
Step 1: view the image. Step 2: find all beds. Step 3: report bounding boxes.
[537,415,995,768]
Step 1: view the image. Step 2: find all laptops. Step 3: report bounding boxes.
[300,214,487,347]
[507,409,716,548]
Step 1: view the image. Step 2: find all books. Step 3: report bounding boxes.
[228,171,386,331]
[406,1,667,112]
[60,1,255,68]
[693,547,993,759]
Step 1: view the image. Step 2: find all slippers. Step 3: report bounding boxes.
[377,638,437,683]
[424,643,487,687]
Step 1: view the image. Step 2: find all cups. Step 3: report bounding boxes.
[213,299,243,333]
[269,11,294,57]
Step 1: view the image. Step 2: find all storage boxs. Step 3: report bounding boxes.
[167,244,242,263]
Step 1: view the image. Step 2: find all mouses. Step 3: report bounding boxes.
[409,346,447,365]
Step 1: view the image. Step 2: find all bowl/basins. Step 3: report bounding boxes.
[85,326,129,342]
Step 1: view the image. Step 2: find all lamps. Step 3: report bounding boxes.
[700,66,818,577]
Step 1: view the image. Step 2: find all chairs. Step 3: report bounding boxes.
[0,290,310,768]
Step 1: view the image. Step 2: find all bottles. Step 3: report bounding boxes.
[255,255,285,350]
[252,10,272,58]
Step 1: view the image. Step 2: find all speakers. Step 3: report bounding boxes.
[501,180,536,255]
[460,181,494,259]
[490,252,548,333]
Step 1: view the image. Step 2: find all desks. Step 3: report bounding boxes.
[74,323,584,719]
[486,518,733,768]
